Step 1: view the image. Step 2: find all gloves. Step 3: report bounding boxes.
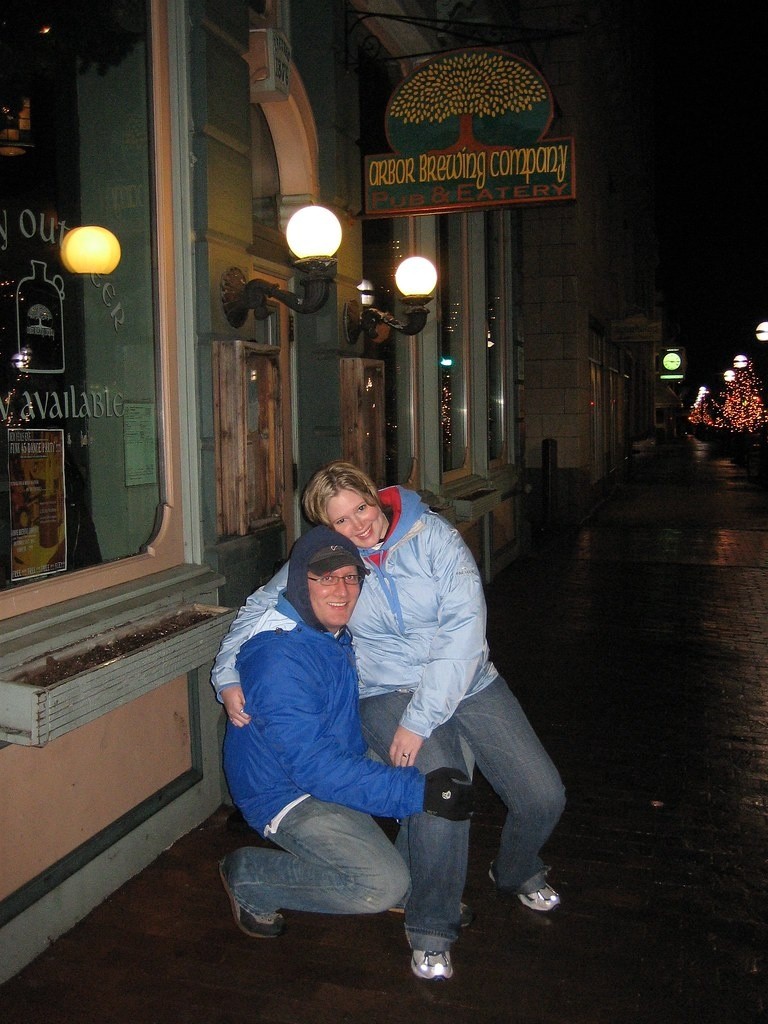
[423,767,479,821]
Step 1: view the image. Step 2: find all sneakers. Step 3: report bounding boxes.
[488,860,562,911]
[218,856,286,938]
[389,902,474,928]
[411,949,454,982]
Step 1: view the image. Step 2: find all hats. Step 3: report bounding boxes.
[309,545,370,577]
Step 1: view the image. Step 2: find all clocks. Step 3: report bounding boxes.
[663,353,681,370]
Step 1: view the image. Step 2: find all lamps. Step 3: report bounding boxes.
[346,255,438,343]
[59,226,121,278]
[221,205,343,329]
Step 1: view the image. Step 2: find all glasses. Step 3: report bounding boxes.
[308,575,364,586]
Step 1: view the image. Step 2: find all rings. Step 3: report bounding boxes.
[403,754,411,758]
[240,707,244,712]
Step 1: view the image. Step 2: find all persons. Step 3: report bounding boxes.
[209,458,568,985]
[220,524,476,940]
